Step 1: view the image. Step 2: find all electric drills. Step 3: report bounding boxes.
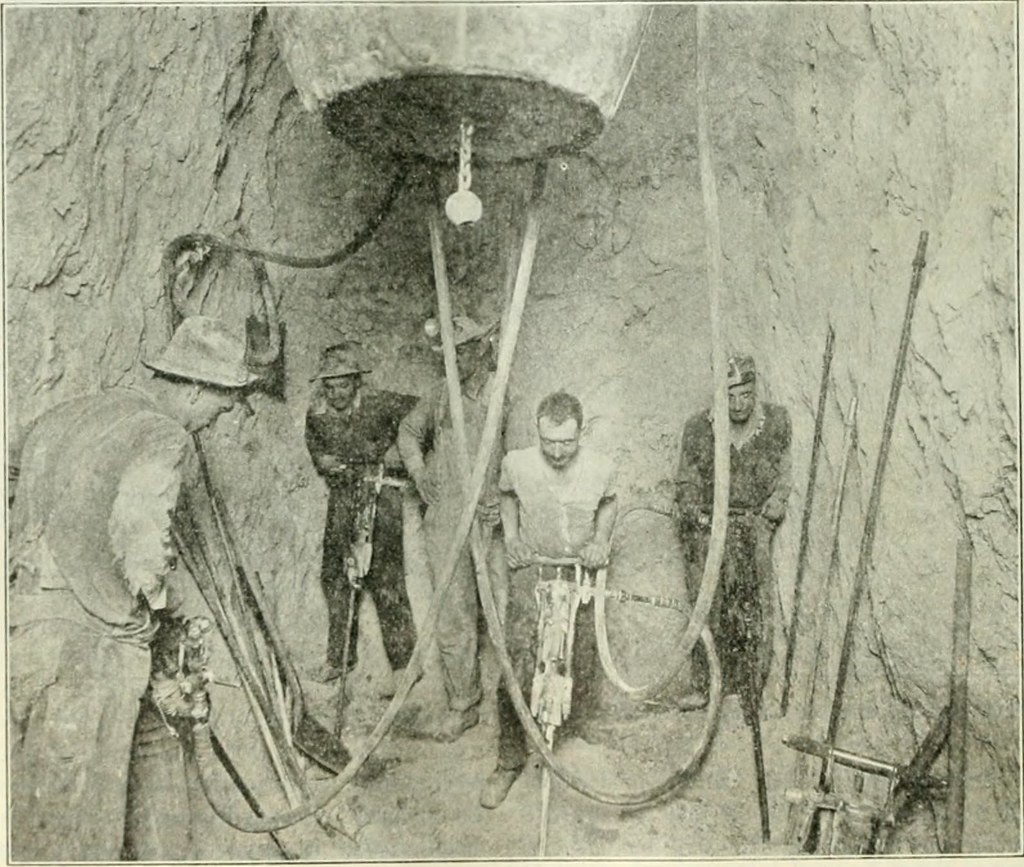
[325,458,392,741]
[692,501,783,844]
[520,558,583,857]
[143,606,302,860]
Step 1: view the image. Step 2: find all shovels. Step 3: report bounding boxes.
[213,489,361,785]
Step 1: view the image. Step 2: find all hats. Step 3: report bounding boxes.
[310,344,372,380]
[729,354,755,388]
[140,316,258,388]
[429,314,500,351]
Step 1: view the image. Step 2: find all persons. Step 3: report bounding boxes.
[400,316,507,740]
[305,346,434,696]
[479,392,619,808]
[677,354,793,708]
[8,317,257,861]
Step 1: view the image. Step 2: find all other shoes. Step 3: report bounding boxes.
[482,767,517,807]
[677,693,706,712]
[379,670,424,696]
[432,708,480,742]
[314,665,354,682]
